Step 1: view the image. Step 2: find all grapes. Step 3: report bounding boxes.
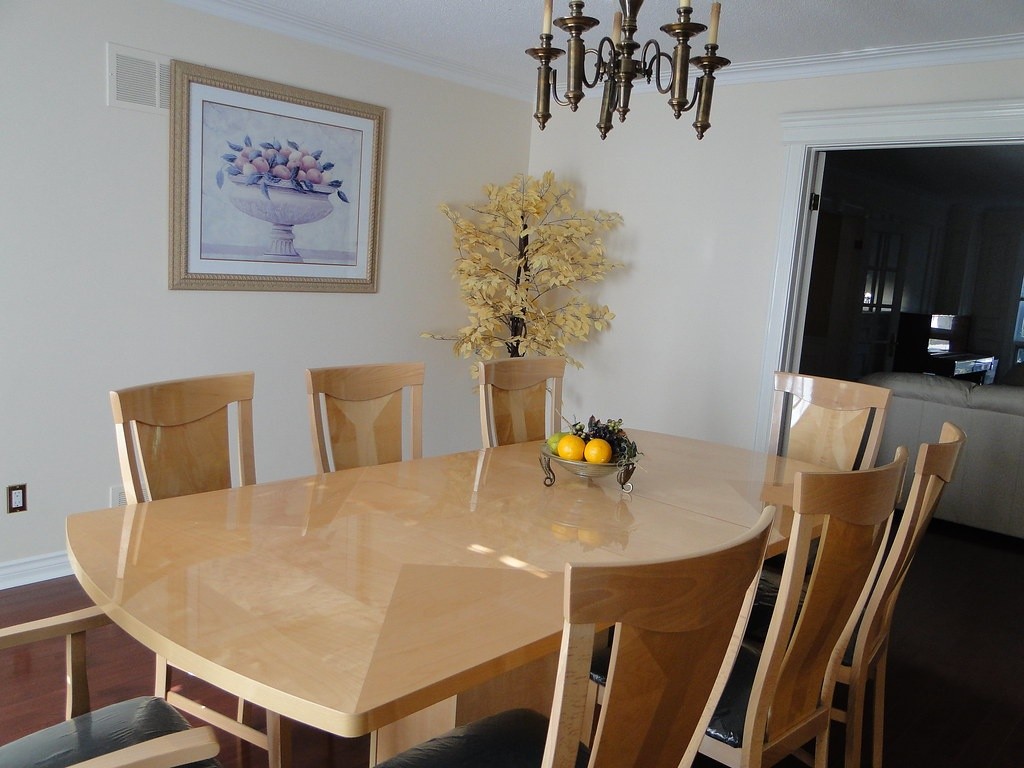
[571,419,626,455]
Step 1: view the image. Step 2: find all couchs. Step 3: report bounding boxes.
[856,370,1024,542]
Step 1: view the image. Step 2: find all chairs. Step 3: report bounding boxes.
[373,503,777,767]
[0,605,230,768]
[306,363,424,471]
[739,422,965,768]
[764,368,887,567]
[112,372,337,768]
[476,356,568,454]
[588,447,904,767]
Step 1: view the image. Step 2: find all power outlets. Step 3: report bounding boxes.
[6,485,29,514]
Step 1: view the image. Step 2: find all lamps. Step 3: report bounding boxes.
[528,0,726,143]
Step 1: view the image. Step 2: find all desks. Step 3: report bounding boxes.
[65,433,853,768]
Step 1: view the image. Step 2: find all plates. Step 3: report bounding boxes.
[540,444,629,478]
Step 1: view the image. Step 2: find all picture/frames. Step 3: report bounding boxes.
[166,60,391,295]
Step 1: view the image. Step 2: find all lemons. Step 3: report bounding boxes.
[546,432,612,463]
